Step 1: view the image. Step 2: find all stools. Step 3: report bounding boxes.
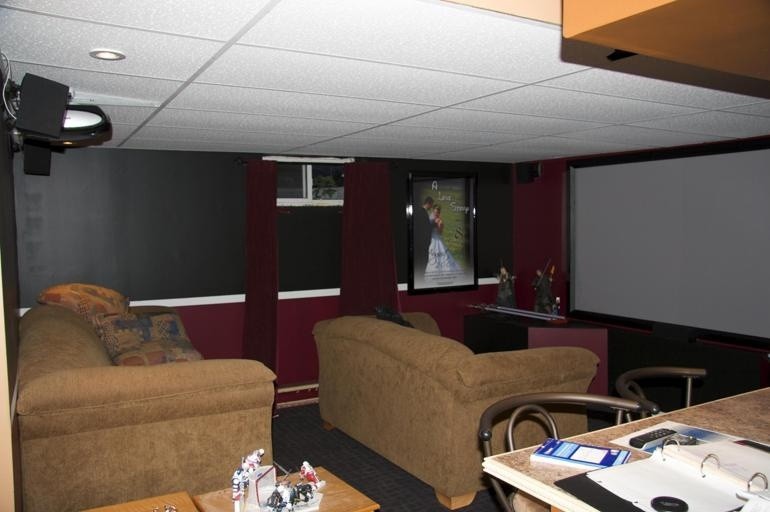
[85,466,380,512]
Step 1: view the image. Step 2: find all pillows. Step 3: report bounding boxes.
[36,281,130,322]
[101,313,204,366]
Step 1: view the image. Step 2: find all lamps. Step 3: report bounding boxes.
[0,68,114,154]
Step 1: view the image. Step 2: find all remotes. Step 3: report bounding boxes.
[629,428,678,451]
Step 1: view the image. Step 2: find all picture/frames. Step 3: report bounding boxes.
[407,169,481,297]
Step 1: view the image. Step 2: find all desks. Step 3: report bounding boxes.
[465,311,609,397]
[482,386,770,512]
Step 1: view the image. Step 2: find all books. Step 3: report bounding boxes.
[740,490,770,512]
[530,436,631,472]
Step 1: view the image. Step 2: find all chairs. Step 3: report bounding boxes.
[479,364,708,512]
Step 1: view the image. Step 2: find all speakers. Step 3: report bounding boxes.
[13,72,72,139]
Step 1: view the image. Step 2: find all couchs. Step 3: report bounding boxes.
[312,311,600,508]
[14,305,277,509]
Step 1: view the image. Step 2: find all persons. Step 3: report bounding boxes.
[494,263,519,308]
[410,197,467,289]
[532,265,563,314]
